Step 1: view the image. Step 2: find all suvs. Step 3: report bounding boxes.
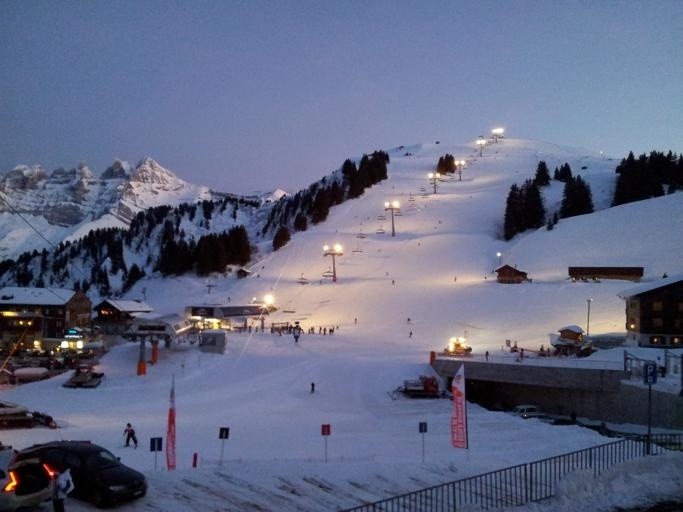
[0,447,55,512]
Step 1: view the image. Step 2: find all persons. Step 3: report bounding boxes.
[123,424,137,447]
[293,322,301,342]
[48,468,76,512]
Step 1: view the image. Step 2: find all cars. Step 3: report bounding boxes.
[545,416,586,429]
[26,440,145,507]
[511,405,546,419]
[0,345,95,372]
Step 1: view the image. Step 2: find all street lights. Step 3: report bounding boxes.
[584,297,594,337]
[322,244,343,282]
[477,140,485,157]
[455,160,466,181]
[429,173,441,194]
[496,252,502,265]
[257,294,275,331]
[385,202,400,237]
[493,129,503,143]
